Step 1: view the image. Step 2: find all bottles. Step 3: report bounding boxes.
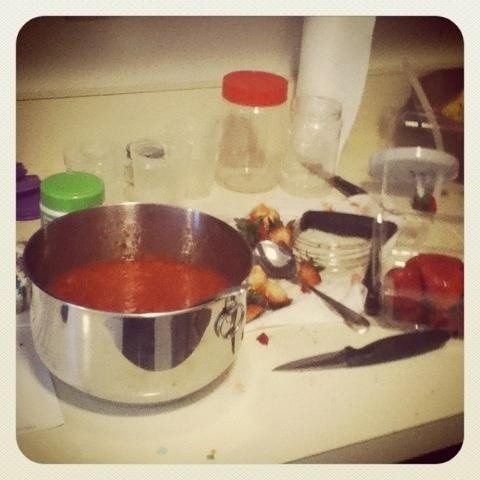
[130,136,182,207]
[213,70,292,194]
[62,137,128,206]
[39,172,106,228]
[277,96,343,200]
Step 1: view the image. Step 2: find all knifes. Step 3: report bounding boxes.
[297,147,367,206]
[275,330,450,371]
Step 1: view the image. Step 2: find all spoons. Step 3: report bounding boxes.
[254,240,369,336]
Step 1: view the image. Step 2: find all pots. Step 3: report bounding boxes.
[15,204,254,404]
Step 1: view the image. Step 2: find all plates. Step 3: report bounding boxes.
[294,232,372,271]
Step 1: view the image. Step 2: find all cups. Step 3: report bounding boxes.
[126,137,180,199]
[63,143,116,195]
